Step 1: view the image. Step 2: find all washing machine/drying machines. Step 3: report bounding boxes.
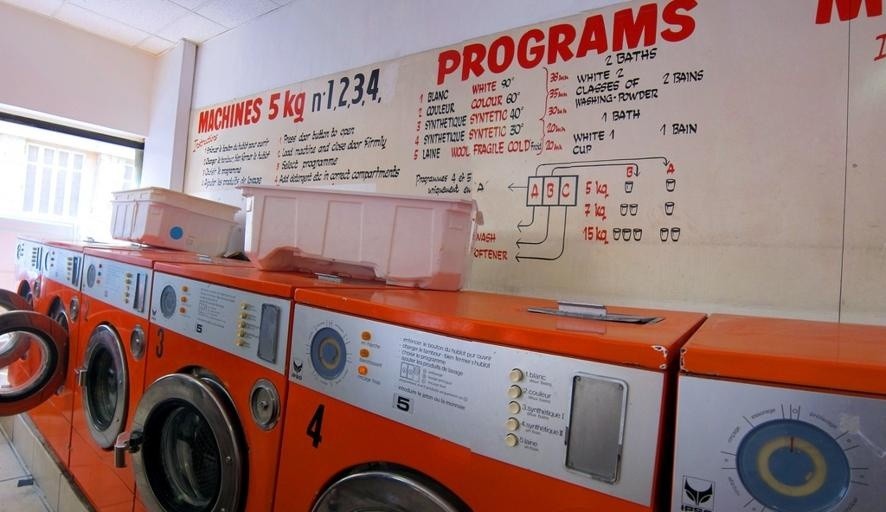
[0,240,886,511]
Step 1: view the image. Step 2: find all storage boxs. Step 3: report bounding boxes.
[240,181,478,293]
[112,186,241,258]
